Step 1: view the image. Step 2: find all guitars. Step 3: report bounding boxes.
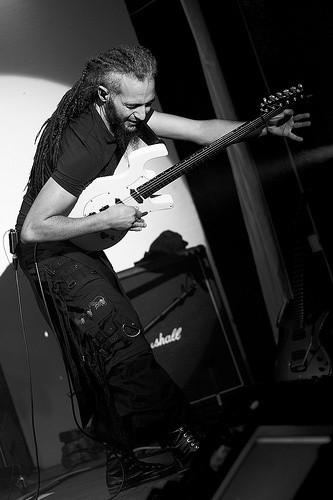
[66,84,314,253]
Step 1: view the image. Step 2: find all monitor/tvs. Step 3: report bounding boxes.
[69,246,258,425]
[211,424,333,500]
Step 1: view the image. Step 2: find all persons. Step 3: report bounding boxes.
[14,44,310,493]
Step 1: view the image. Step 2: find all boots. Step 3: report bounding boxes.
[106,441,175,494]
[163,422,200,478]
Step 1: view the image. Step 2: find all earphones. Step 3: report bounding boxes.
[100,90,107,98]
